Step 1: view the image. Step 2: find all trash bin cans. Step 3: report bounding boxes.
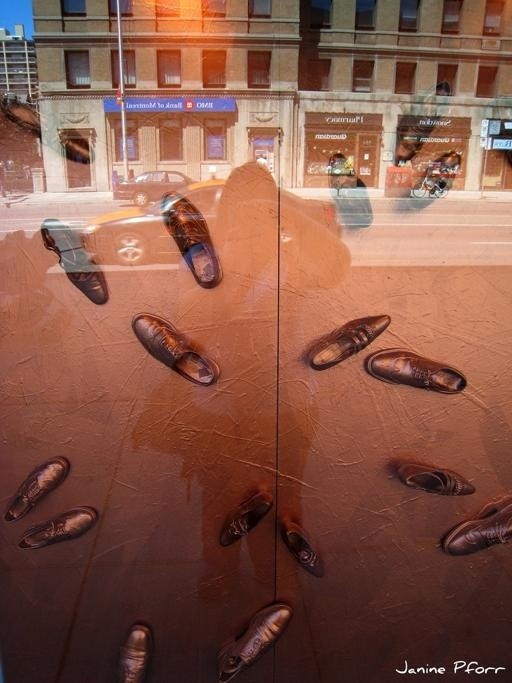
[384,167,411,198]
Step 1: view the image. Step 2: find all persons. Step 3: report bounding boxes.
[153,157,354,598]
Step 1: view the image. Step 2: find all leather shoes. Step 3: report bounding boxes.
[116,622,155,682]
[161,191,225,291]
[16,504,101,553]
[394,78,451,165]
[281,519,324,579]
[303,315,396,372]
[2,454,71,526]
[327,151,376,228]
[405,149,462,211]
[40,214,114,305]
[215,602,295,683]
[218,492,276,548]
[364,347,468,396]
[1,90,98,166]
[392,458,477,498]
[130,310,221,388]
[438,494,511,557]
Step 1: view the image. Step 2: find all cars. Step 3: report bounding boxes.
[109,168,203,211]
[78,183,345,271]
